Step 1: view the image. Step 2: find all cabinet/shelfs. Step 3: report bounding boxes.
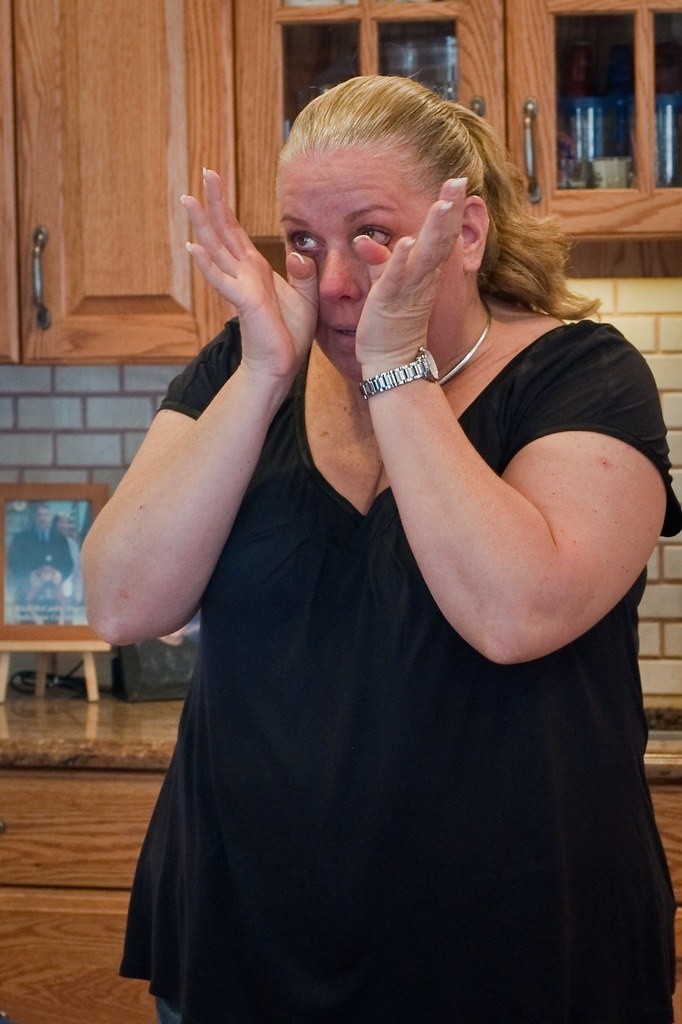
[0,0,682,365]
[1,769,681,1024]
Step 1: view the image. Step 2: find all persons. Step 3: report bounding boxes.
[78,75,682,1024]
[8,507,78,625]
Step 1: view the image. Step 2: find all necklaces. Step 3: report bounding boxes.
[438,300,491,385]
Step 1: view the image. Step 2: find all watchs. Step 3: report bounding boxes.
[359,346,440,398]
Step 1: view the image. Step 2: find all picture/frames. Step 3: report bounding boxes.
[0,484,110,640]
[121,609,203,703]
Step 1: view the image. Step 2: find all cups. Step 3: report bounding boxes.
[561,159,590,187]
[590,156,632,188]
